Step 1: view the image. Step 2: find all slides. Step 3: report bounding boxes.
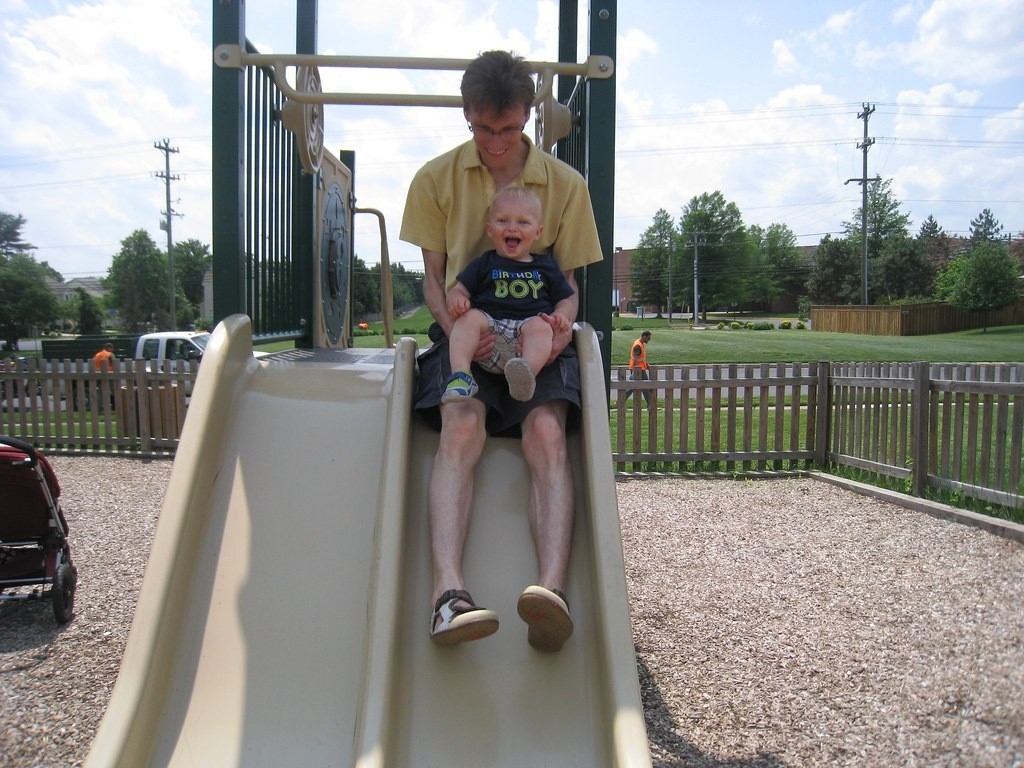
[83,309,655,768]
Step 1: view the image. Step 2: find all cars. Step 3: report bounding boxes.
[0,356,41,399]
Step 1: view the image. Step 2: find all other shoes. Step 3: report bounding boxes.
[428,588,499,648]
[443,370,478,404]
[503,359,535,402]
[516,583,574,656]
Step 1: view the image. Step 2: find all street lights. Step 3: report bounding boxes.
[614,246,622,317]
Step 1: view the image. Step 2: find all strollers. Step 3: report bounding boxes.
[0,435,78,622]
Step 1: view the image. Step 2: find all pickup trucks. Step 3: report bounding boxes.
[45,330,270,412]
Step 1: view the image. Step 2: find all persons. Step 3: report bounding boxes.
[93,343,116,411]
[441,183,573,402]
[625,330,651,411]
[400,51,603,652]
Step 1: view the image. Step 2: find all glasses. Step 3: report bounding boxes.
[646,336,651,341]
[463,113,529,140]
